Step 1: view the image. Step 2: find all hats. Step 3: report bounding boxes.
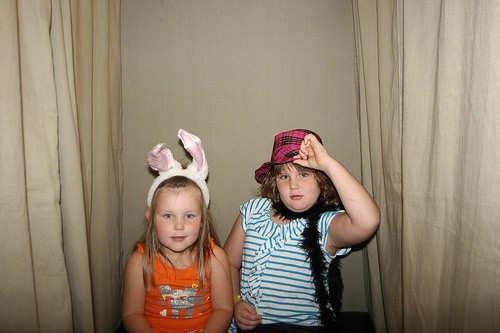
[255,129,324,184]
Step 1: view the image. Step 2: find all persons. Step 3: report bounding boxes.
[222,128,381,332]
[115,128,236,333]
